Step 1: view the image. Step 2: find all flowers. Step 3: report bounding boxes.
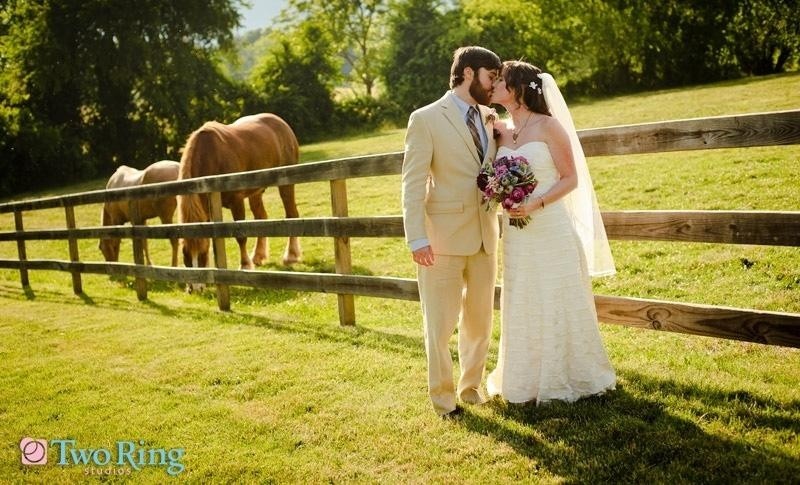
[476,156,539,230]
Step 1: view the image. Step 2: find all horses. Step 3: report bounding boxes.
[99,160,180,267]
[177,112,301,295]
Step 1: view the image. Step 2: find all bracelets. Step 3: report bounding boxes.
[539,195,544,208]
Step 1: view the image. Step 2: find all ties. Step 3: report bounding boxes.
[466,107,483,163]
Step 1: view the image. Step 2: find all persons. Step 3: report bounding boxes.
[400,45,503,422]
[486,59,618,404]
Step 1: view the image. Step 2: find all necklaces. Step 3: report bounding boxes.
[511,110,533,144]
